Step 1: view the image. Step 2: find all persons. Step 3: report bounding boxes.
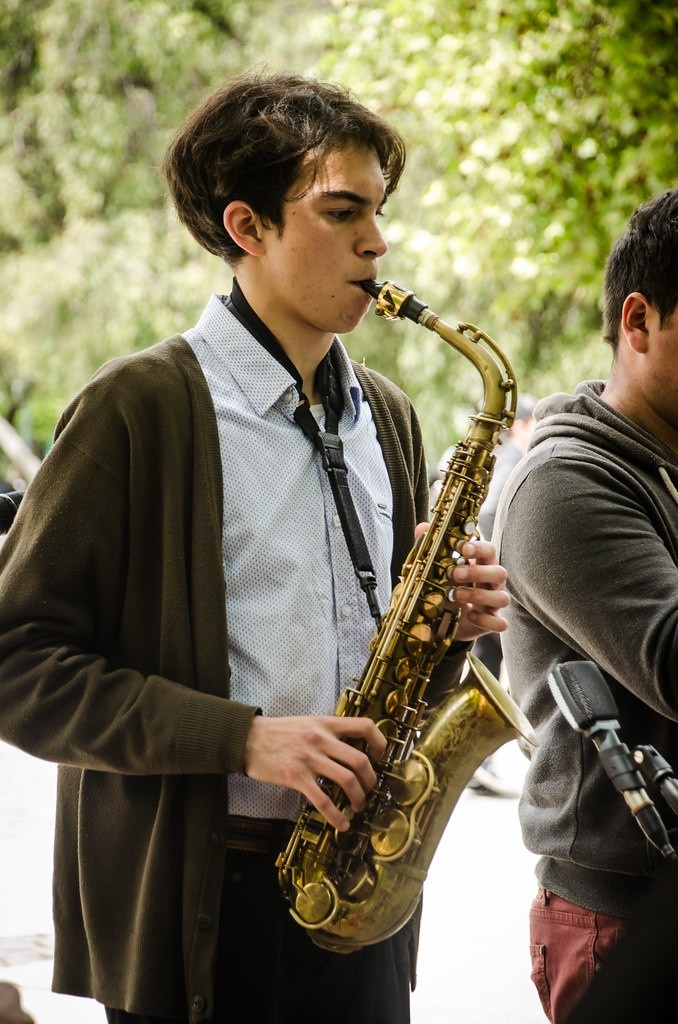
[468,394,533,799]
[0,79,515,1024]
[481,187,678,1024]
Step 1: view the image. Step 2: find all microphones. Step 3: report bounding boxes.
[547,660,674,859]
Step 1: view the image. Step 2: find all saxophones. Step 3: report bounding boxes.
[270,280,543,956]
